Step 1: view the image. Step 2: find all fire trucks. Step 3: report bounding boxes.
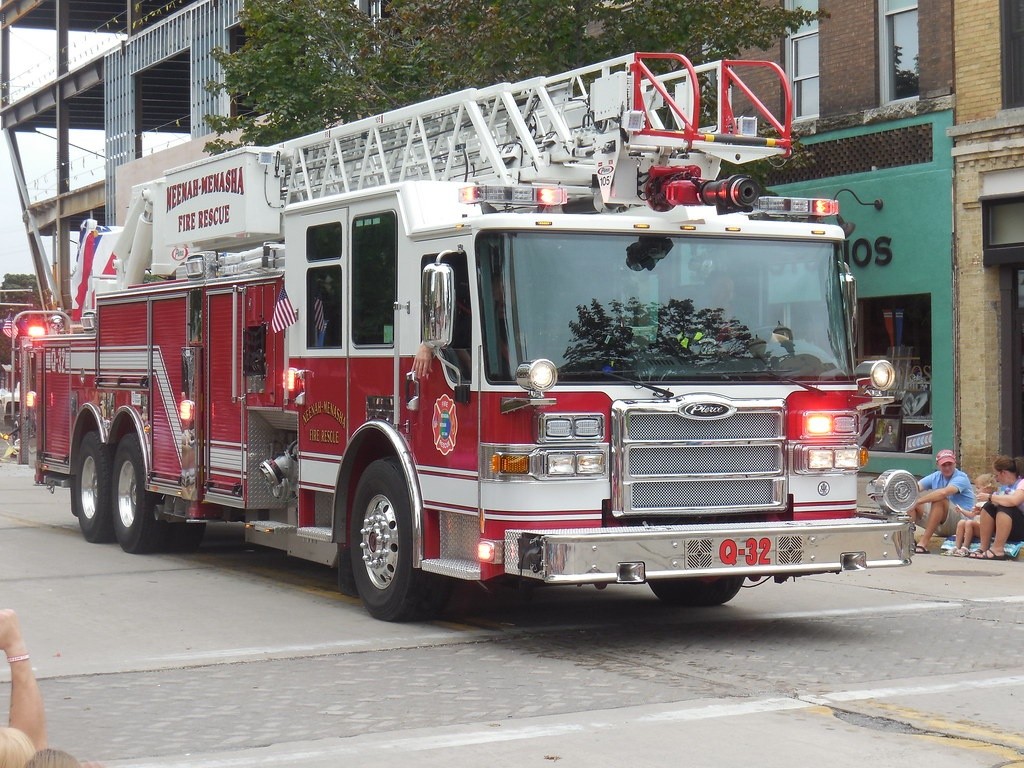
[2,50,919,623]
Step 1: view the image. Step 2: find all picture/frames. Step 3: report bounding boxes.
[869,415,903,452]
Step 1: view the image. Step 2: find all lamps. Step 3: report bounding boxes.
[834,188,883,239]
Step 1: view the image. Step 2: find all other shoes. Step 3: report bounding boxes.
[954,547,970,556]
[976,549,1006,559]
[945,546,958,555]
[969,549,983,558]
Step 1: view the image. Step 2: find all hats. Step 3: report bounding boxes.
[936,450,956,466]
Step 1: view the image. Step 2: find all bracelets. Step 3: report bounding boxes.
[988,494,992,503]
[7,654,30,662]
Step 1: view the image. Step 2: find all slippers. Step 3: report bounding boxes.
[915,546,929,554]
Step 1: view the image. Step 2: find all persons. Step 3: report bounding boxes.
[969,455,1024,560]
[411,271,533,381]
[944,473,998,557]
[0,608,81,768]
[908,450,974,553]
[676,270,790,356]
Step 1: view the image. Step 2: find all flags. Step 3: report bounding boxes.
[3,308,19,339]
[314,297,324,332]
[271,281,299,333]
